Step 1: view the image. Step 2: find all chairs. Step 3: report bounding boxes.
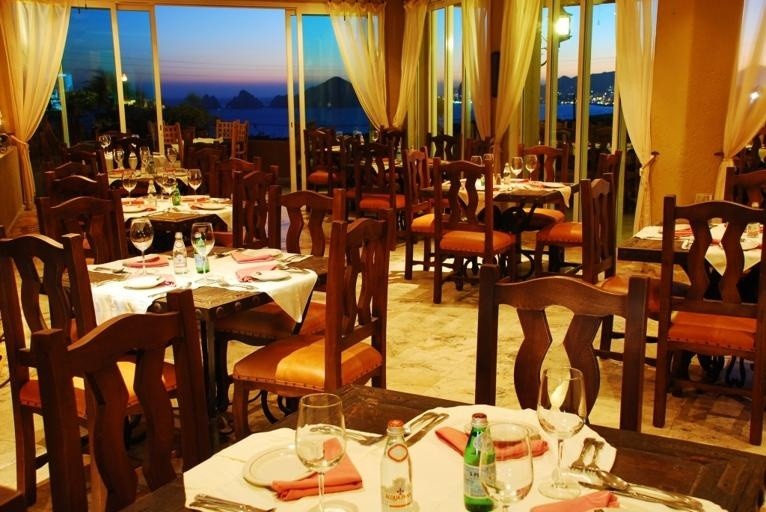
[31,288,204,512]
[403,147,478,279]
[654,195,766,444]
[232,208,387,453]
[181,145,227,194]
[40,159,90,197]
[210,155,263,247]
[722,167,766,222]
[353,141,406,250]
[53,175,100,199]
[333,134,364,219]
[475,264,650,433]
[433,159,517,303]
[176,127,195,162]
[58,142,103,160]
[582,173,691,387]
[69,150,97,175]
[381,127,407,155]
[304,127,348,215]
[426,133,456,161]
[517,144,569,271]
[227,121,249,161]
[105,152,168,169]
[96,128,132,153]
[231,165,287,248]
[201,187,343,436]
[38,190,126,265]
[156,122,184,160]
[465,136,494,165]
[0,227,180,503]
[217,120,233,141]
[535,150,621,273]
[315,127,336,146]
[109,135,143,169]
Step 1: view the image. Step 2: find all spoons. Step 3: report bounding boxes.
[594,468,703,510]
[402,413,435,437]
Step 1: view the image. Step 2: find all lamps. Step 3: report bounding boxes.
[551,0,572,41]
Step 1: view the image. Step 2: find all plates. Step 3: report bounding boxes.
[251,270,290,281]
[242,445,320,487]
[719,239,759,251]
[242,248,281,256]
[466,419,529,444]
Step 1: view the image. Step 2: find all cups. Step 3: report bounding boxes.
[482,153,495,170]
[478,422,534,512]
[525,154,538,183]
[295,393,346,512]
[112,148,123,168]
[694,193,713,206]
[162,173,177,212]
[190,221,216,285]
[124,277,165,289]
[153,167,168,205]
[194,202,225,210]
[99,135,108,154]
[511,156,524,184]
[129,217,155,276]
[537,367,588,498]
[470,155,482,165]
[167,147,178,173]
[188,168,202,204]
[105,135,112,156]
[122,173,138,208]
[140,146,151,176]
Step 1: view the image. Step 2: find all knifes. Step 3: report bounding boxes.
[405,413,449,449]
[579,482,699,510]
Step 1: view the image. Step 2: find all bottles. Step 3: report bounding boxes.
[381,420,412,512]
[397,146,401,162]
[496,173,501,185]
[504,163,510,190]
[410,146,414,154]
[360,136,364,145]
[148,180,157,201]
[464,414,495,512]
[194,234,209,273]
[172,233,187,274]
[748,202,760,239]
[171,183,180,206]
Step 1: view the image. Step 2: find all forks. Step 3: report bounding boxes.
[194,494,274,511]
[311,429,377,446]
[582,440,603,473]
[217,278,254,290]
[568,437,596,472]
[313,422,383,442]
